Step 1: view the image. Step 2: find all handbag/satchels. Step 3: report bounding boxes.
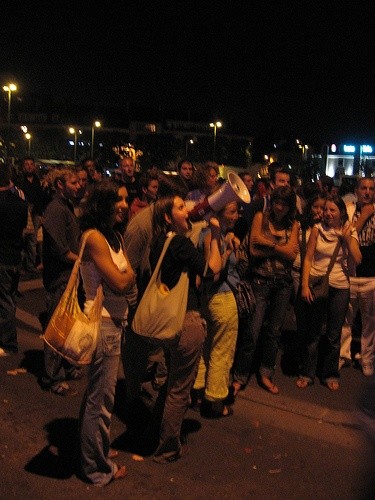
[298,273,329,300]
[43,230,107,366]
[234,280,257,315]
[132,232,191,339]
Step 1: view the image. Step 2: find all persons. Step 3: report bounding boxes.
[0,146,375,487]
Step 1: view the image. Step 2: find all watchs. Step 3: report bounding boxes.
[270,243,275,251]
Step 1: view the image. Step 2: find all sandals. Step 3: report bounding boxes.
[339,357,345,370]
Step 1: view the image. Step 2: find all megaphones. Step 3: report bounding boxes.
[188,171,251,226]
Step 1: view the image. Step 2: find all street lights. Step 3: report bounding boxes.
[2,82,17,122]
[209,121,222,158]
[25,133,32,156]
[69,127,77,161]
[91,120,101,160]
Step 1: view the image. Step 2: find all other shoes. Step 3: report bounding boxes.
[47,382,77,396]
[296,377,308,390]
[326,379,339,389]
[260,377,279,395]
[200,406,233,418]
[361,359,374,376]
